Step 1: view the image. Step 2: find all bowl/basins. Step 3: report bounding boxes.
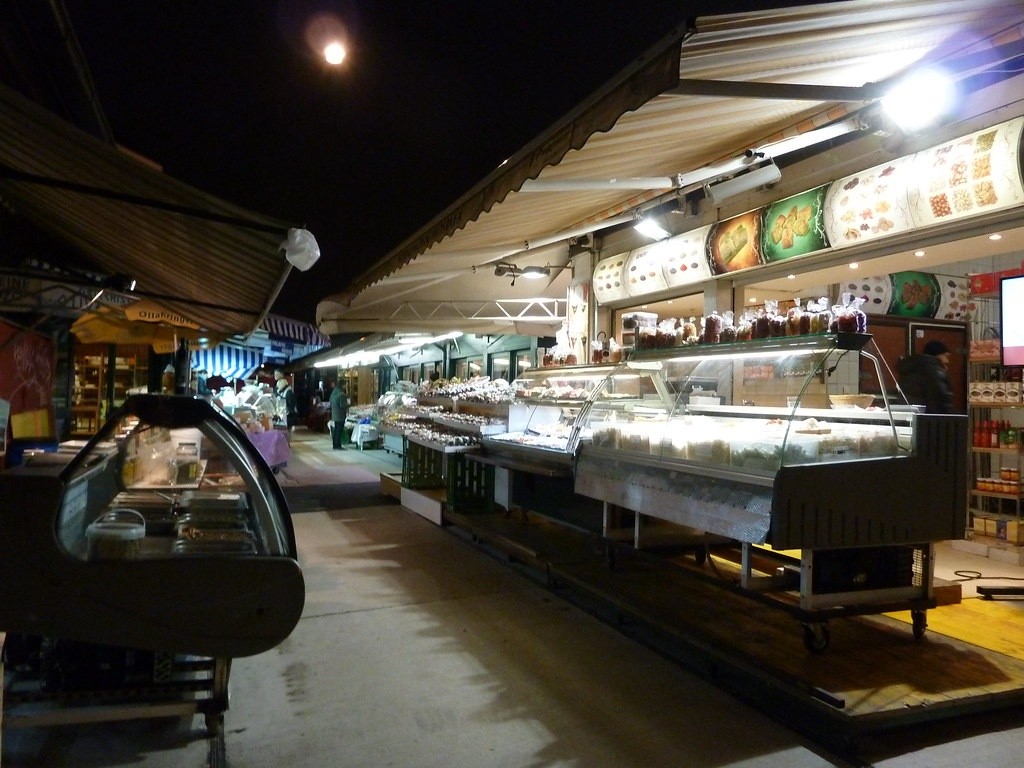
[828,394,877,409]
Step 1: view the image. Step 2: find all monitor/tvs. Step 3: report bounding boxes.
[999,274,1024,368]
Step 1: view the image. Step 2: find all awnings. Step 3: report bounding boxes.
[0,1,1024,375]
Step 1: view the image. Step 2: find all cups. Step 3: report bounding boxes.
[786,396,802,409]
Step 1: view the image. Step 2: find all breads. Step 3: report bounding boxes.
[771,205,812,248]
[515,386,607,399]
[901,280,930,311]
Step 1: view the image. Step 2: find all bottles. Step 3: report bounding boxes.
[974,419,1024,449]
[161,336,208,395]
[176,442,198,484]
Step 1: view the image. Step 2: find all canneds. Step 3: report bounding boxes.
[977,466,1024,494]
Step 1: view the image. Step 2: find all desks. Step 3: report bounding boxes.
[0,440,121,557]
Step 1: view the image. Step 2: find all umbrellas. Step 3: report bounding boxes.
[71,292,226,365]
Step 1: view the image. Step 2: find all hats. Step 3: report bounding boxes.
[923,340,948,356]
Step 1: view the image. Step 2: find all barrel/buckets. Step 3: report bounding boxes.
[84,508,146,563]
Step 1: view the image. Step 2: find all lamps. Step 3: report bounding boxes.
[631,210,673,243]
[521,264,576,280]
[702,157,782,209]
[108,273,138,294]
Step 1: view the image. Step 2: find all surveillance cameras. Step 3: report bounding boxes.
[745,149,756,157]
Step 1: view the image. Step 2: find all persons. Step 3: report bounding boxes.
[271,369,296,447]
[325,380,352,452]
[896,339,954,411]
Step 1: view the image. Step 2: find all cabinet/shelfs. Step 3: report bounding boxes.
[966,294,1024,549]
